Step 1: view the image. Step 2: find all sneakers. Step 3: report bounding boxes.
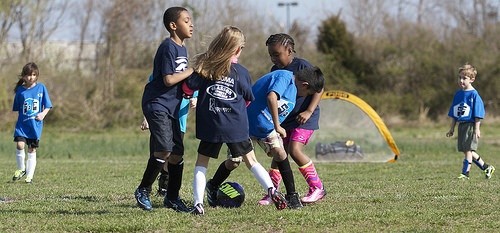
[301,183,326,202]
[134,187,153,209]
[259,191,282,205]
[164,196,190,211]
[284,192,303,210]
[205,179,217,207]
[268,187,287,209]
[187,204,204,215]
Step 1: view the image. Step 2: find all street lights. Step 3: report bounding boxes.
[278,1,298,36]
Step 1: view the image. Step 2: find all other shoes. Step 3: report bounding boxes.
[484,165,495,179]
[155,190,167,198]
[13,170,26,181]
[25,176,33,183]
[457,174,470,180]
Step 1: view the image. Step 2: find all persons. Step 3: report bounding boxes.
[446,64,496,180]
[135,7,326,215]
[12,62,53,183]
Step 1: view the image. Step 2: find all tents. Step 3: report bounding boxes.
[288,90,400,163]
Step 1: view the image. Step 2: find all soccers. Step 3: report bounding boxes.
[217,182,245,207]
